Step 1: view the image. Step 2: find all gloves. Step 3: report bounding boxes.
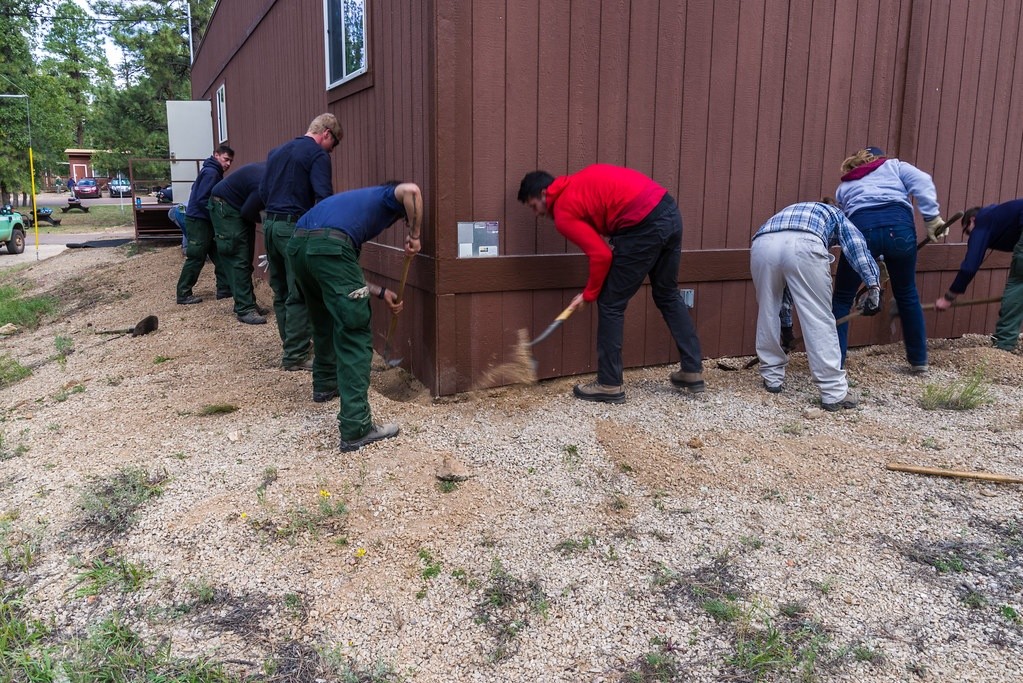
[925,214,950,244]
[780,324,796,353]
[855,287,884,316]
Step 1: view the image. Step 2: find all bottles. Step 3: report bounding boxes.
[136,198,141,208]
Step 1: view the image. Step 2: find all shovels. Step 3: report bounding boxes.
[371,256,414,370]
[855,211,964,315]
[716,309,864,370]
[523,304,578,369]
[95,315,158,337]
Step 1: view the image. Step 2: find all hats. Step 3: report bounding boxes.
[865,146,885,157]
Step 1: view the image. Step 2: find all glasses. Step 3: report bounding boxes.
[324,127,340,150]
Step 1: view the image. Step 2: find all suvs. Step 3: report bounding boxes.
[0,205,30,254]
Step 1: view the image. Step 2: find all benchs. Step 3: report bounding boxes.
[61,199,90,213]
[23,209,61,229]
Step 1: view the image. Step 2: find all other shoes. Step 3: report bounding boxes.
[237,311,265,325]
[286,353,316,371]
[310,340,315,354]
[177,295,202,304]
[215,285,234,299]
[911,359,929,371]
[256,306,270,316]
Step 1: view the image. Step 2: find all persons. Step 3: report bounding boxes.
[936,198,1023,352]
[258,113,344,371]
[177,145,237,304]
[750,197,881,411]
[517,163,705,404]
[56,176,76,197]
[288,180,424,452]
[207,161,269,323]
[832,146,950,371]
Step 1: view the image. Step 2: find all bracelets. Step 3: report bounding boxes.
[409,232,420,240]
[378,287,386,300]
[944,292,954,301]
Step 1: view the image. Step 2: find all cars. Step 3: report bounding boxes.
[73,177,102,199]
[108,178,132,197]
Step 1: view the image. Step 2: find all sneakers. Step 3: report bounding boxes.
[313,385,340,403]
[573,377,625,403]
[340,423,399,453]
[670,369,705,393]
[822,392,856,412]
[763,378,782,392]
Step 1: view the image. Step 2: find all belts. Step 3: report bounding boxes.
[210,194,230,208]
[292,229,354,248]
[267,214,302,223]
